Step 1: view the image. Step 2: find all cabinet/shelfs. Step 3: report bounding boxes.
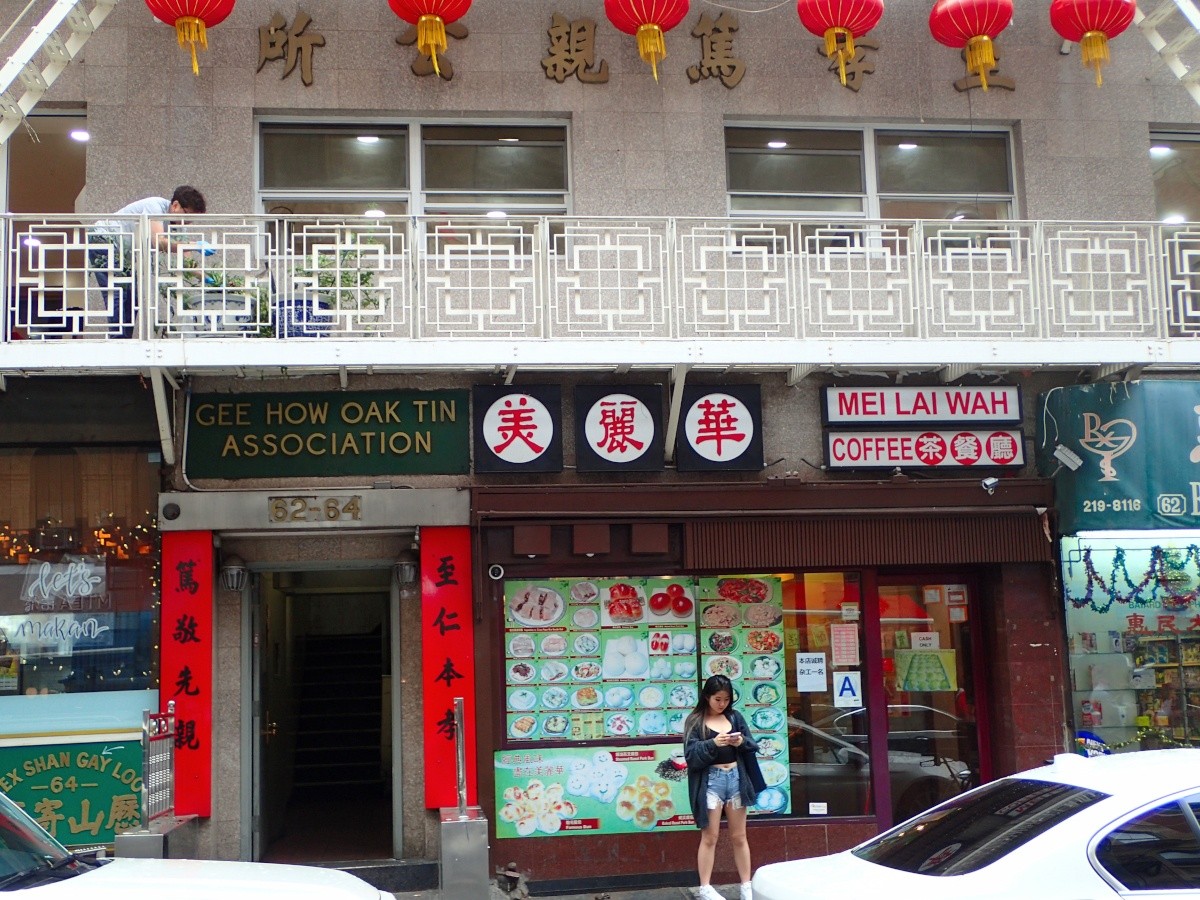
[1132,634,1200,748]
[1069,651,1155,729]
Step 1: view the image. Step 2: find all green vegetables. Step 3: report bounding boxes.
[736,579,763,603]
[718,660,735,675]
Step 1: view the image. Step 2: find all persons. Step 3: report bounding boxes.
[88,185,216,339]
[684,675,760,900]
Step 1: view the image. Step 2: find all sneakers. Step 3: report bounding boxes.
[699,884,726,900]
[740,881,753,900]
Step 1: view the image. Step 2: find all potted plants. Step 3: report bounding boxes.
[88,228,391,338]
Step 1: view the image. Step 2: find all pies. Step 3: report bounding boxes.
[514,717,535,733]
[509,589,556,621]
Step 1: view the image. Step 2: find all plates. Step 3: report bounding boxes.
[508,582,697,737]
[701,577,783,707]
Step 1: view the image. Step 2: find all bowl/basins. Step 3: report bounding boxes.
[748,656,789,814]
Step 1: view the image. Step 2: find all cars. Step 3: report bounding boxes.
[751,747,1199,897]
[787,702,980,768]
[0,789,397,900]
[747,716,975,820]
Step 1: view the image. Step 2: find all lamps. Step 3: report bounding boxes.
[393,548,420,590]
[221,553,248,593]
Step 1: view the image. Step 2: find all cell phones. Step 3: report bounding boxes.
[729,731,742,741]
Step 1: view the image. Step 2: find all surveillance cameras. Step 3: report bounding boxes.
[982,477,999,490]
[1053,445,1084,471]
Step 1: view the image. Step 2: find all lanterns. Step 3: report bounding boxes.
[798,0,884,87]
[145,0,235,75]
[604,0,690,81]
[1050,0,1137,89]
[929,0,1013,90]
[388,0,473,76]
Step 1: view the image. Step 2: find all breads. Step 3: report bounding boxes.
[609,584,642,622]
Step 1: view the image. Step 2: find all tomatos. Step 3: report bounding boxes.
[648,584,692,618]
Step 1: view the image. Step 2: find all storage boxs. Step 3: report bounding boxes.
[1081,700,1101,727]
